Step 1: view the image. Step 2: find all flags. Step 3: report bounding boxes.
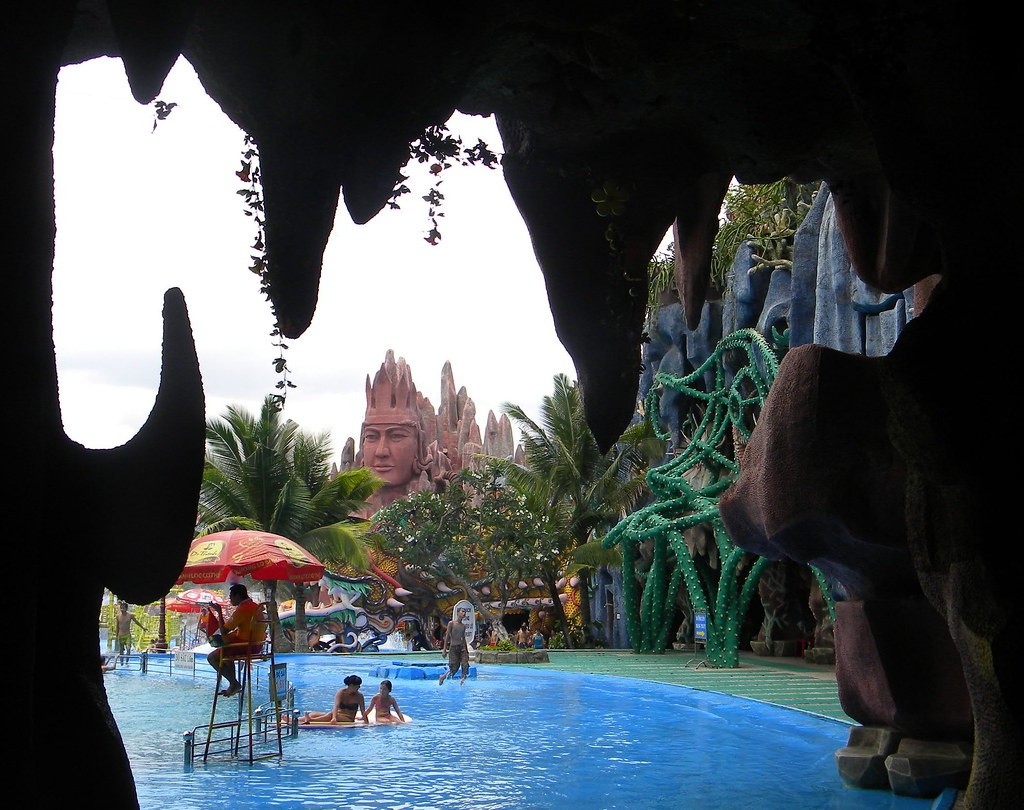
[206,611,220,637]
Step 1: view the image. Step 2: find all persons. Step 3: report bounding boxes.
[197,608,209,633]
[101,653,120,673]
[207,584,266,698]
[281,675,369,724]
[116,603,148,666]
[360,407,433,520]
[515,623,531,651]
[531,627,544,649]
[405,633,413,641]
[355,680,404,723]
[439,607,470,685]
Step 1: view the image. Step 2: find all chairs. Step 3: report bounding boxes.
[203,601,282,766]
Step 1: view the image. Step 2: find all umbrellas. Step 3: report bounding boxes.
[166,588,228,613]
[174,530,325,585]
